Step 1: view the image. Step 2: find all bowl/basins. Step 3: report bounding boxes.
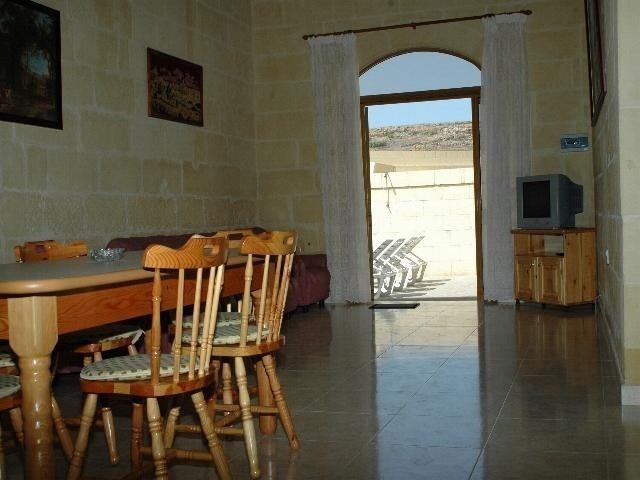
[89,248,128,263]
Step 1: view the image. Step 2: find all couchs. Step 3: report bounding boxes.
[106,228,332,324]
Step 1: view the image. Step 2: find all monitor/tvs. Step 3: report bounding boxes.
[516,174,584,230]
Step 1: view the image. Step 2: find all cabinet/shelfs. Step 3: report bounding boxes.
[509,226,598,313]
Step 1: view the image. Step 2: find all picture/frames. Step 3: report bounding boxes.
[583,0,608,128]
[147,48,203,127]
[0,0,63,130]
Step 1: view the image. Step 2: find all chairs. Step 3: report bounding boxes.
[371,235,428,301]
